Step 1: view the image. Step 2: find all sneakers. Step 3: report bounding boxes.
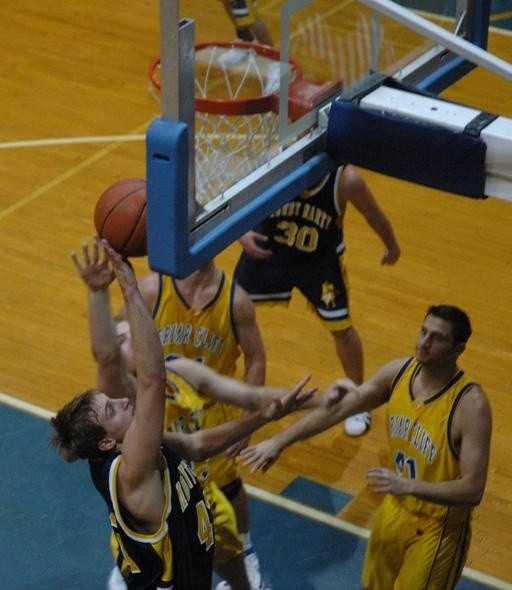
[243,543,261,590]
[219,39,257,65]
[109,566,128,590]
[262,61,280,97]
[345,412,371,436]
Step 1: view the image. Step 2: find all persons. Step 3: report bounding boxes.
[68,242,360,590]
[51,235,319,589]
[235,304,492,590]
[114,256,266,588]
[233,163,400,437]
[216,1,262,65]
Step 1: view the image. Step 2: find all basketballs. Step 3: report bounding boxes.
[95,178,147,258]
